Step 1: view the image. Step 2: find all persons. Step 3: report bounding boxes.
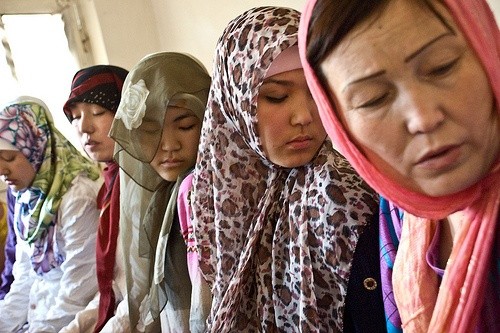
[297,0,500,333]
[58,66,128,333]
[97,50,212,333]
[1,96,102,333]
[178,5,380,333]
